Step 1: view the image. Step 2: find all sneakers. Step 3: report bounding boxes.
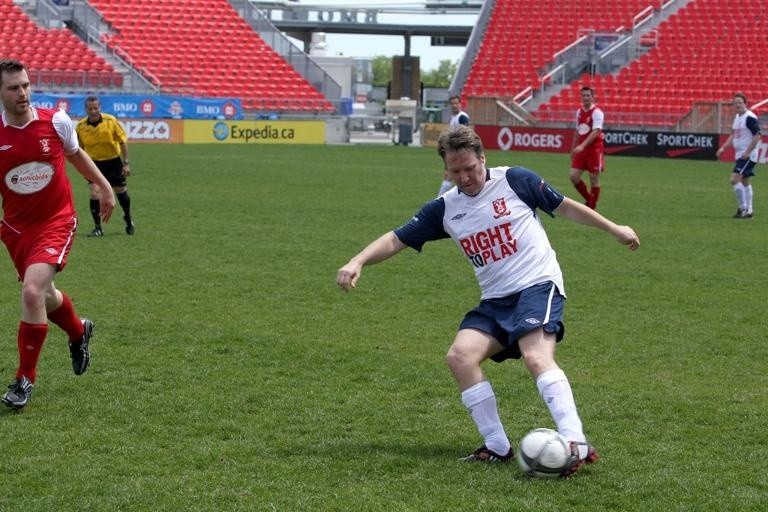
[2,375,34,408]
[457,444,514,465]
[122,214,135,235]
[68,318,95,376]
[733,208,754,218]
[562,443,599,477]
[88,228,104,236]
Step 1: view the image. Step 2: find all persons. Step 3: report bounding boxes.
[1,58,118,410]
[436,95,471,202]
[568,86,605,212]
[713,94,762,218]
[333,125,642,475]
[73,95,137,236]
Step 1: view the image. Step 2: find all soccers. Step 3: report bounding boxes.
[516,428,572,478]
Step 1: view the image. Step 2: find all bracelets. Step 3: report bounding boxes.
[122,160,129,164]
